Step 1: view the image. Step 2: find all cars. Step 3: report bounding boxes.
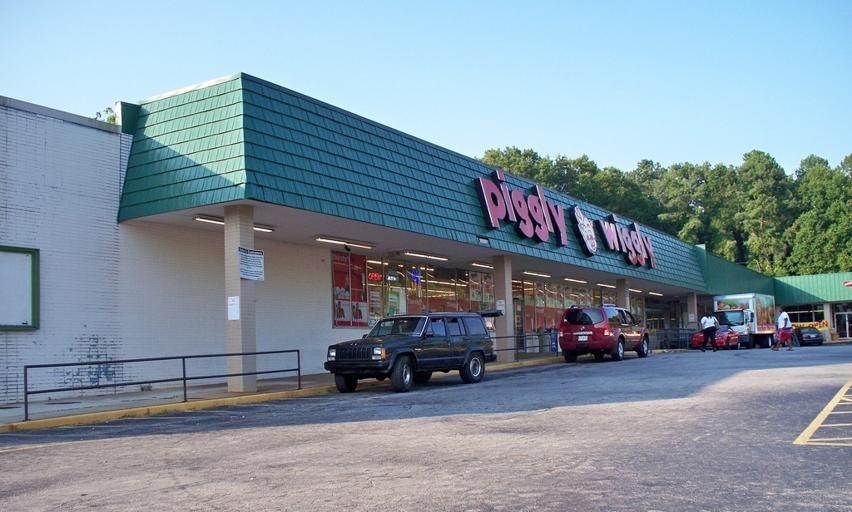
[559,304,650,362]
[795,327,823,346]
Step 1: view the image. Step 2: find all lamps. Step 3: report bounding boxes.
[313,234,377,250]
[565,278,587,284]
[398,251,450,261]
[522,270,551,278]
[191,214,275,233]
[472,261,495,271]
[512,280,591,298]
[368,260,435,271]
[363,281,494,297]
[598,283,616,288]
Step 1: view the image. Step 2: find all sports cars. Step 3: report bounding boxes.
[690,325,742,350]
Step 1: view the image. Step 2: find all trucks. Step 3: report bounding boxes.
[713,293,776,348]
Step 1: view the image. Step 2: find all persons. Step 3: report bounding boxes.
[772,304,794,351]
[699,311,720,352]
[769,307,781,350]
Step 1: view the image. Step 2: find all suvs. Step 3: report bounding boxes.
[324,313,496,393]
